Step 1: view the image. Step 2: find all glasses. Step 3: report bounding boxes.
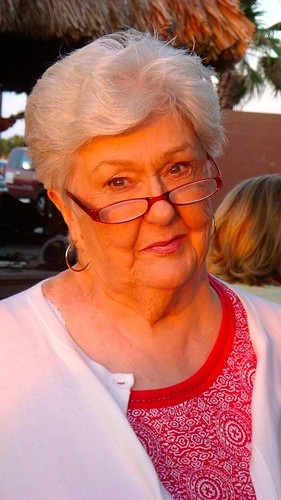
[66,150,223,225]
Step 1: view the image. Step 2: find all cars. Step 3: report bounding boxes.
[2,147,50,215]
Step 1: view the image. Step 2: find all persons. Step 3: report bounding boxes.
[0,29,281,500]
[206,174,281,307]
